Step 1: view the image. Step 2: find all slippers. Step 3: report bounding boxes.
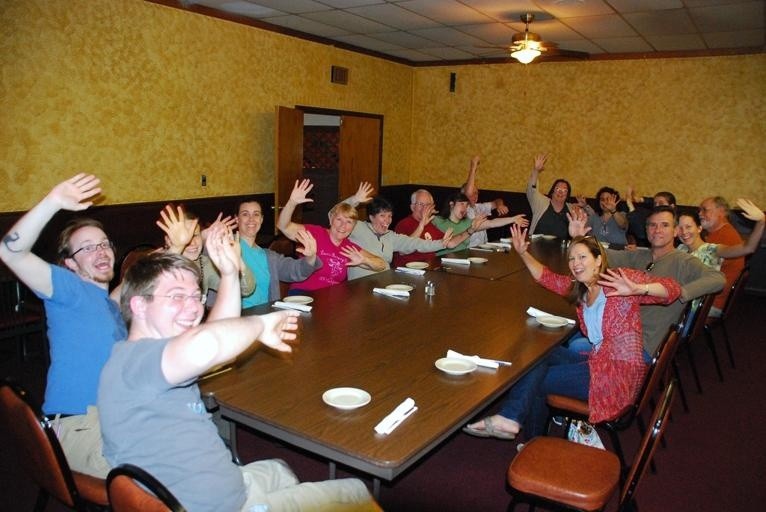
[463,417,515,441]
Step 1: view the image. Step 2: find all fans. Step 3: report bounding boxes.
[471,13,591,58]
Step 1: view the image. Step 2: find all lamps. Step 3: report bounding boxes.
[511,47,542,65]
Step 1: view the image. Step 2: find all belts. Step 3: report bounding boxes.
[46,414,72,420]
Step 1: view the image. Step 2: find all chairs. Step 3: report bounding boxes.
[2,384,112,510]
[684,267,751,371]
[501,381,677,512]
[541,326,682,479]
[103,462,192,512]
[611,300,695,422]
[655,284,724,395]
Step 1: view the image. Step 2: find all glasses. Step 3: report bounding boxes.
[72,242,112,258]
[144,291,206,306]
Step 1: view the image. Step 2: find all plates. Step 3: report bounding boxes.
[282,294,314,306]
[536,315,569,328]
[385,284,414,292]
[467,257,488,264]
[322,387,372,411]
[406,261,430,269]
[542,235,557,240]
[434,357,478,376]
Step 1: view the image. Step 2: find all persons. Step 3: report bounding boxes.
[0,173,129,480]
[461,223,680,453]
[277,178,387,296]
[199,196,324,310]
[565,206,726,365]
[528,153,766,337]
[156,204,257,298]
[100,229,371,512]
[336,154,529,280]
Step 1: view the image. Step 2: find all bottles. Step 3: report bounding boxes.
[424,280,436,295]
